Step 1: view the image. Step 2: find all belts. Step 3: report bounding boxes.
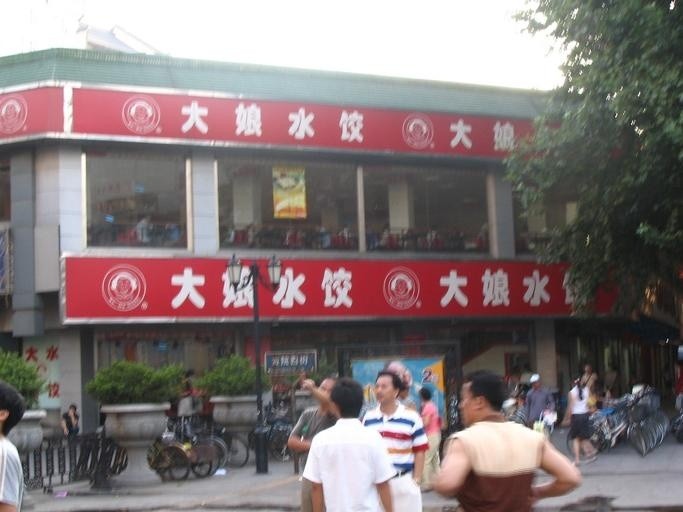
[395,469,408,478]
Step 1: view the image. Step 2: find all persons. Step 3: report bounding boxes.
[390,273,413,298]
[408,118,430,140]
[110,271,138,300]
[431,369,582,511]
[398,381,415,410]
[0,380,28,512]
[560,371,598,467]
[361,368,431,512]
[181,367,195,395]
[383,360,423,391]
[417,387,441,494]
[220,218,358,249]
[671,358,683,414]
[88,213,186,247]
[521,373,555,434]
[60,404,79,446]
[286,376,338,512]
[0,98,20,123]
[129,101,153,123]
[367,223,486,251]
[577,363,601,398]
[300,375,398,512]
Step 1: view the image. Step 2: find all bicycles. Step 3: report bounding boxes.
[248,401,295,462]
[672,396,683,443]
[146,415,250,482]
[566,389,672,457]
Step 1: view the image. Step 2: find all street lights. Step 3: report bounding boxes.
[228,253,282,474]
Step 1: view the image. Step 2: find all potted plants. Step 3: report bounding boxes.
[82,360,189,488]
[0,350,54,512]
[205,354,274,465]
[291,362,340,445]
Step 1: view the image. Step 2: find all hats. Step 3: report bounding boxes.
[502,398,516,408]
[529,374,540,384]
